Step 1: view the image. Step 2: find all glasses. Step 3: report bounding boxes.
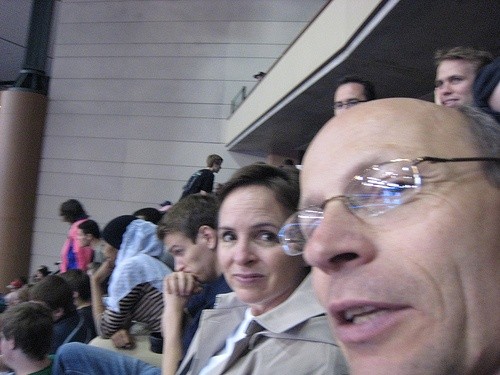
[330,98,368,109]
[278,156,500,256]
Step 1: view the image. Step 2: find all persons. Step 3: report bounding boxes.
[31,274,80,354]
[0,301,56,375]
[58,199,93,273]
[169,163,349,375]
[335,75,374,113]
[133,207,173,270]
[433,46,493,106]
[53,193,231,375]
[475,55,500,114]
[88,214,182,367]
[77,220,115,294]
[179,154,223,199]
[0,265,49,311]
[279,99,500,375]
[57,269,91,312]
